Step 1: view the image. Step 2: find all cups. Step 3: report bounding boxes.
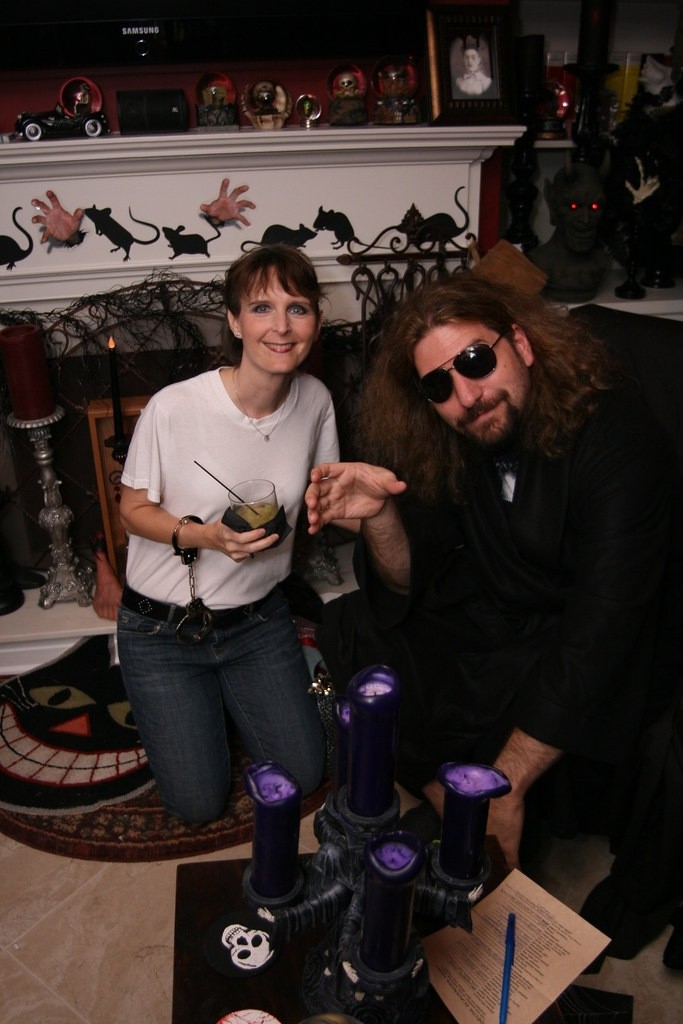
[228,479,278,530]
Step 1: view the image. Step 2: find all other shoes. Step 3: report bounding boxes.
[580,870,671,962]
[0,562,46,590]
[0,583,24,616]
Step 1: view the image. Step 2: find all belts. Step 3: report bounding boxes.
[120,584,276,630]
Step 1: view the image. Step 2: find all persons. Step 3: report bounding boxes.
[92,532,123,621]
[304,274,683,972]
[31,179,256,243]
[116,243,361,823]
[526,163,612,290]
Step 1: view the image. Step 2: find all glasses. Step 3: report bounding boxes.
[419,328,509,405]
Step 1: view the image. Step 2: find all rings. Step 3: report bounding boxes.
[249,552,255,559]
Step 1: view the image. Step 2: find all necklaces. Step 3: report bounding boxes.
[232,366,290,441]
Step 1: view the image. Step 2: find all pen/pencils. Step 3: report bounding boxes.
[499,913,518,1024]
